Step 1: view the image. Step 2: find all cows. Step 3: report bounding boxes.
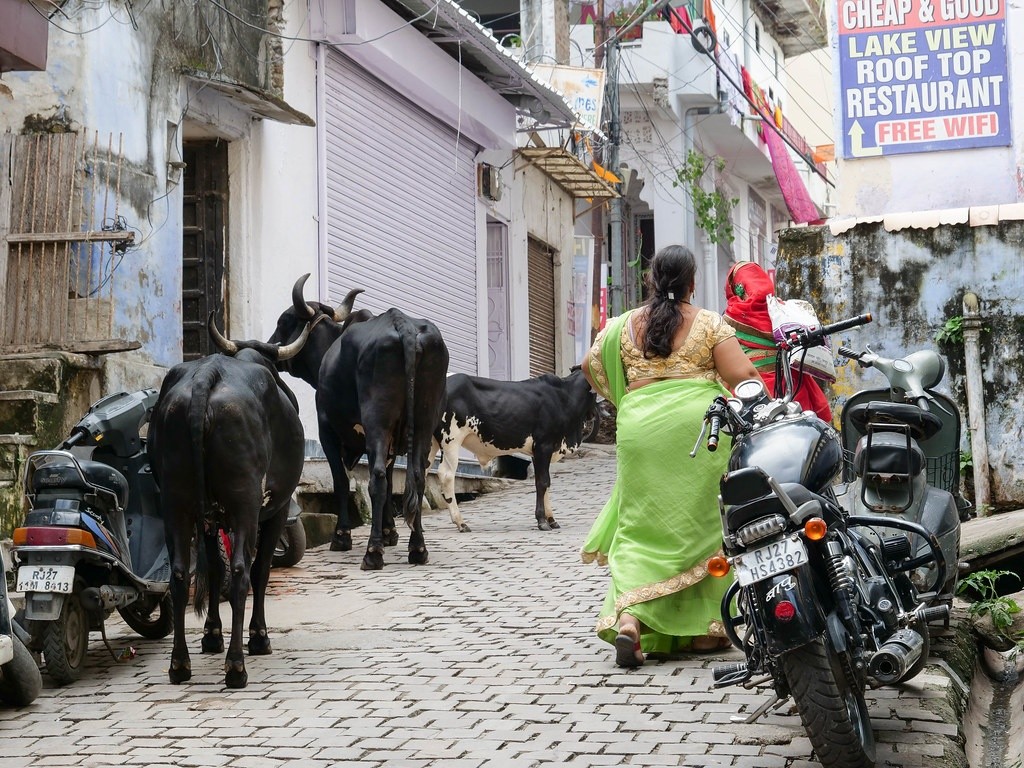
[146,273,606,690]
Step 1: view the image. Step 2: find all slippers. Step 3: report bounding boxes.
[692,637,732,654]
[614,635,641,668]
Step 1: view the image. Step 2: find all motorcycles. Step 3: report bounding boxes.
[0,543,43,706]
[689,313,950,767]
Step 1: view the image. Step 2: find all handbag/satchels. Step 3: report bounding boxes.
[767,293,836,384]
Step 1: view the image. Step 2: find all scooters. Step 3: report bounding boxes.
[838,346,970,628]
[268,493,308,569]
[15,387,177,686]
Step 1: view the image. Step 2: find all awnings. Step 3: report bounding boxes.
[516,146,623,222]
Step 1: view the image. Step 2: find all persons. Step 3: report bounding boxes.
[716,260,835,429]
[581,245,775,670]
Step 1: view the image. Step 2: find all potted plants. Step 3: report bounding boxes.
[604,0,645,39]
